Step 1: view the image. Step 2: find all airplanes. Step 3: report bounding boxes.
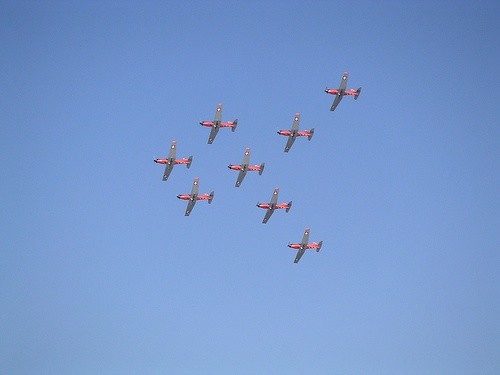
[288,227,323,263]
[324,71,362,111]
[177,176,214,216]
[154,140,193,181]
[227,147,266,187]
[277,112,315,152]
[200,103,238,144]
[256,187,293,224]
